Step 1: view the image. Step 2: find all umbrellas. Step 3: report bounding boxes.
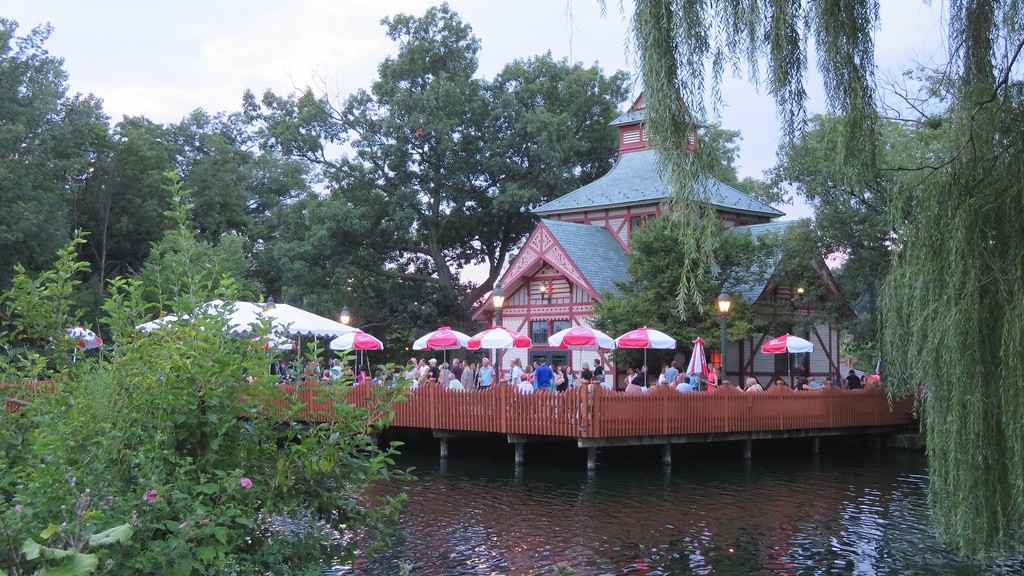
[248,333,296,350]
[761,333,814,387]
[328,330,383,383]
[413,327,471,377]
[47,325,103,363]
[686,337,709,391]
[548,326,615,379]
[466,326,533,383]
[614,326,677,386]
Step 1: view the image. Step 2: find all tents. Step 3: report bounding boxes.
[186,299,365,382]
[135,311,190,333]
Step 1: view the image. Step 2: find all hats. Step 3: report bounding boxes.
[428,358,437,363]
[849,369,854,373]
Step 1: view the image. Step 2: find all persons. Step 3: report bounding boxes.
[507,358,613,423]
[242,356,365,386]
[370,357,502,392]
[623,361,718,393]
[735,364,879,396]
